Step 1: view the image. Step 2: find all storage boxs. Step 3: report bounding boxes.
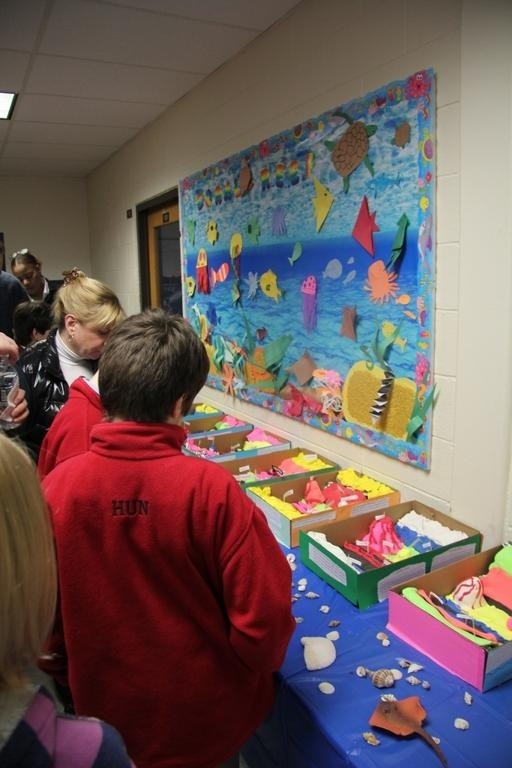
[246,466,402,550]
[215,446,341,491]
[383,539,512,694]
[297,499,487,612]
[185,423,292,464]
[179,402,226,435]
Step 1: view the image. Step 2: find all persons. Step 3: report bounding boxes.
[40,311,297,768]
[2,433,139,768]
[1,248,126,477]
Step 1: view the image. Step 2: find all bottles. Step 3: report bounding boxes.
[0,354,21,431]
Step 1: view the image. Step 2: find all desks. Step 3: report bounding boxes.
[258,538,512,765]
